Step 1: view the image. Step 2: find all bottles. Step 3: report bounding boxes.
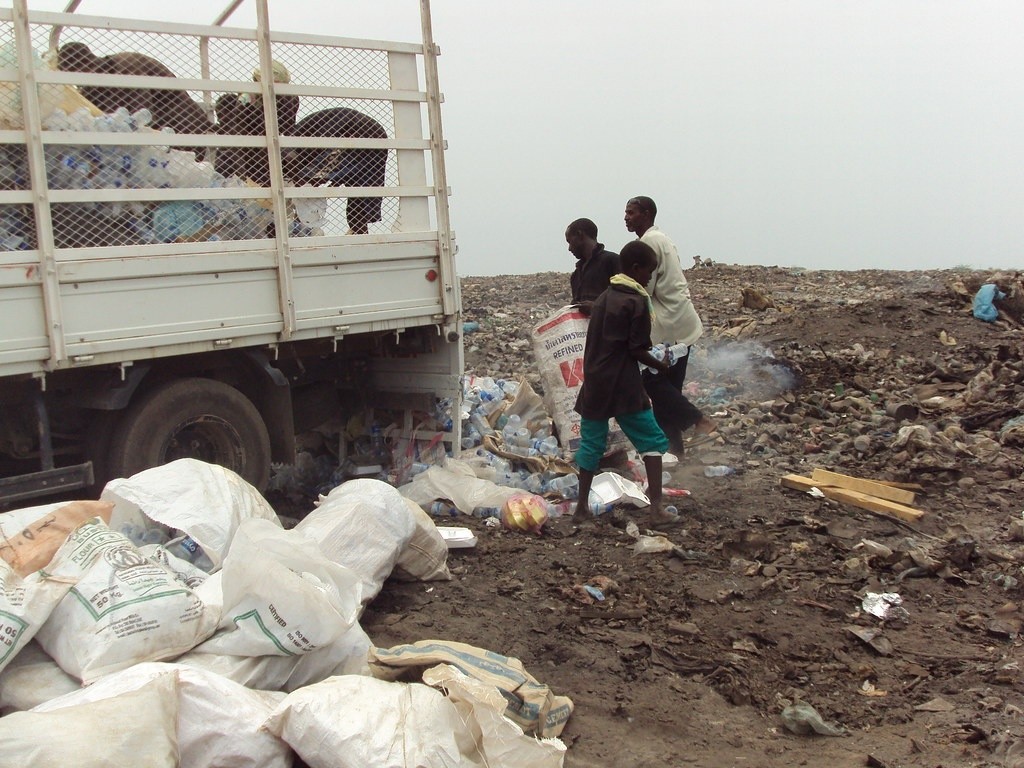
[410,375,612,517]
[0,107,312,252]
[628,464,692,516]
[116,510,213,571]
[704,465,737,478]
[582,584,606,602]
[637,342,690,375]
[312,424,391,496]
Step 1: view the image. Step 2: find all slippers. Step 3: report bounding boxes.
[655,514,688,530]
[665,456,689,470]
[572,511,600,525]
[683,434,722,449]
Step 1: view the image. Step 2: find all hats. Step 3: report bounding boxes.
[253,57,291,84]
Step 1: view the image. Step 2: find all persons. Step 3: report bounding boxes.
[57,41,209,164]
[618,195,721,461]
[570,241,689,533]
[564,217,620,315]
[215,59,389,234]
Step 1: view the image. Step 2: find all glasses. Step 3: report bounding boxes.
[626,197,647,213]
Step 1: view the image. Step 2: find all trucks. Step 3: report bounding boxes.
[0,0,467,504]
